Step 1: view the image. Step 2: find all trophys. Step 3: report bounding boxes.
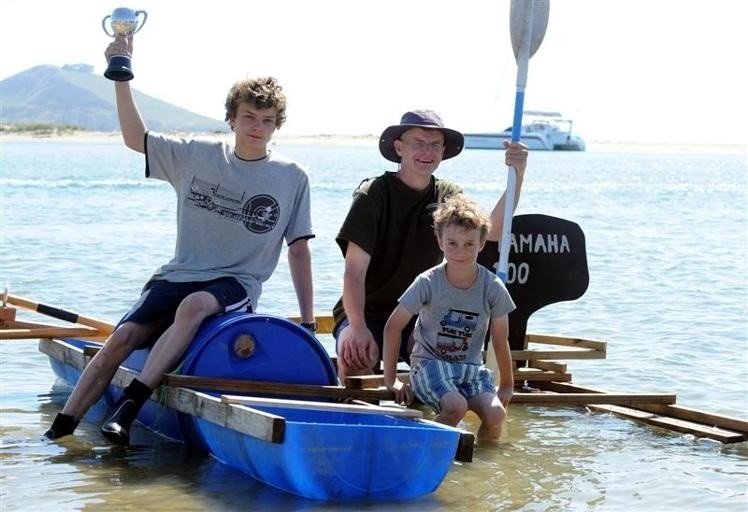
[102,7,148,82]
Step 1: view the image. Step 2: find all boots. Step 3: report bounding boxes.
[40,411,80,441]
[100,377,154,445]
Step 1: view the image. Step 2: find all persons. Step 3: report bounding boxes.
[327,108,530,388]
[3,33,319,463]
[380,194,517,440]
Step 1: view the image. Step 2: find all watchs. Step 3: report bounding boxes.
[299,322,318,331]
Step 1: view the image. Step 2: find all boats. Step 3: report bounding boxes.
[464,111,585,151]
[35,322,461,500]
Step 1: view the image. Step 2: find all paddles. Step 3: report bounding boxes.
[487,0,549,395]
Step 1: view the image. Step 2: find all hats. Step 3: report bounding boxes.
[378,109,465,164]
